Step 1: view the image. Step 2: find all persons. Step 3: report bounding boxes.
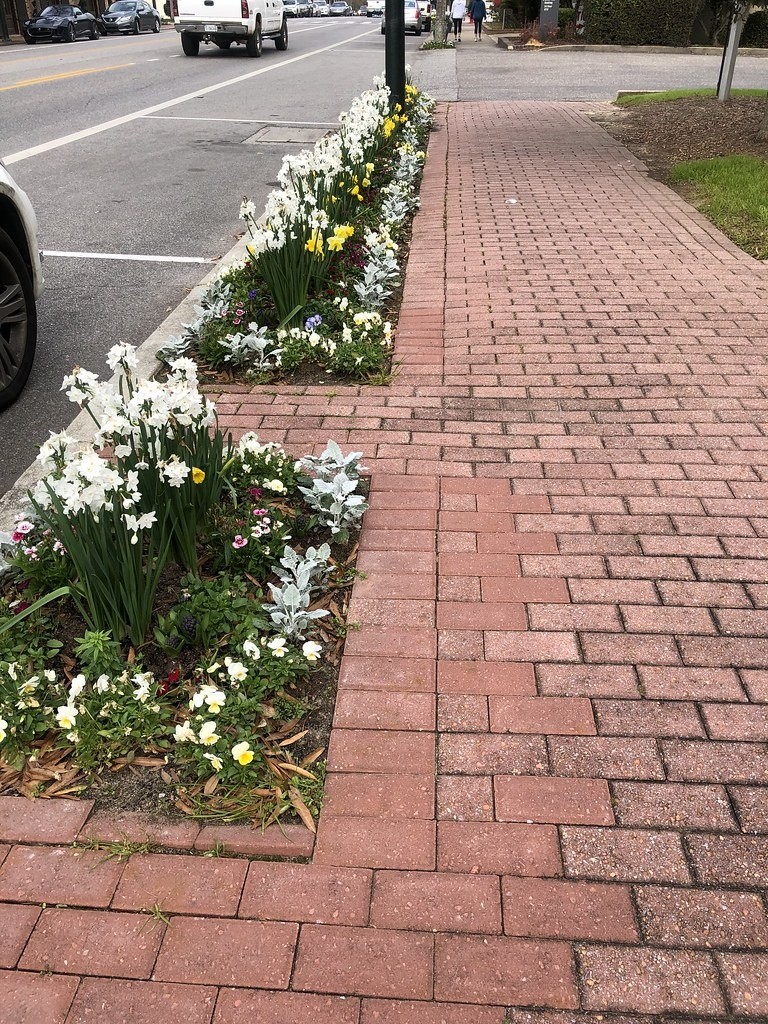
[451,0,467,41]
[470,0,486,42]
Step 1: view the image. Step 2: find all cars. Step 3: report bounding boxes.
[1,160,45,412]
[97,0,161,36]
[284,0,435,35]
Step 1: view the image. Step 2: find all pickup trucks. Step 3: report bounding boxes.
[171,0,289,58]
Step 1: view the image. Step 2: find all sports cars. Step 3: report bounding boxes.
[23,4,98,43]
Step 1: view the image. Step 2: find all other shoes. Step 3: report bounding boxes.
[455,38,482,42]
